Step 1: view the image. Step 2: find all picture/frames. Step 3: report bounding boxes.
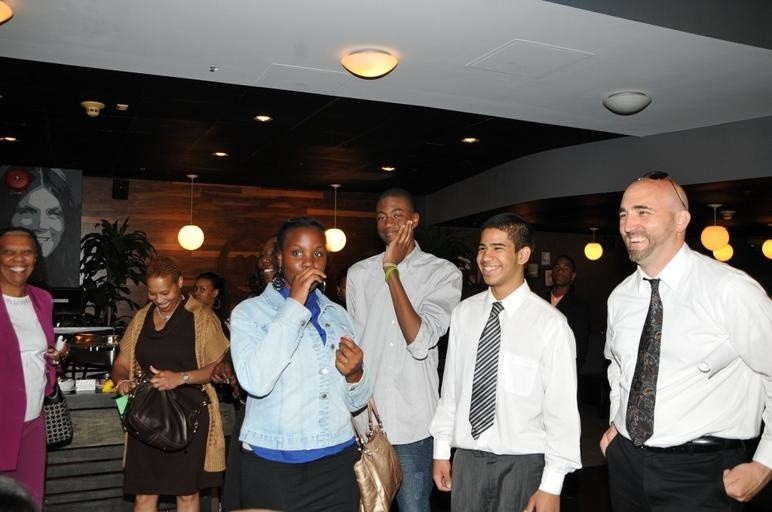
[524,251,554,288]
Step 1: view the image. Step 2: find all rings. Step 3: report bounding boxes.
[344,358,348,364]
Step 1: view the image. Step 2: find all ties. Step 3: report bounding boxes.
[469,302,504,440]
[626,278,662,447]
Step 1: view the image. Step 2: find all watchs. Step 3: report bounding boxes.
[182,371,190,385]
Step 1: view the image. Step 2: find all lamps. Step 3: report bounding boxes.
[339,47,398,79]
[323,184,347,252]
[0,1,14,23]
[178,174,205,250]
[583,227,604,261]
[701,204,772,262]
[602,90,652,116]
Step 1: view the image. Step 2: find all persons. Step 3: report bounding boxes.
[210,235,285,509]
[231,214,375,511]
[190,271,227,310]
[2,167,76,259]
[0,226,60,510]
[427,211,585,511]
[344,188,463,511]
[598,169,772,511]
[111,256,231,511]
[539,253,586,372]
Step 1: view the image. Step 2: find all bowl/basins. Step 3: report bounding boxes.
[58,379,74,389]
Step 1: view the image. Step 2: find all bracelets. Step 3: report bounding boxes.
[115,379,130,391]
[383,262,400,283]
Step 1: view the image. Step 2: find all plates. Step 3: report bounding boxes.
[59,388,73,394]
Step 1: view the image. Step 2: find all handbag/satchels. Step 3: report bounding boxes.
[44,385,73,448]
[123,373,198,451]
[351,396,402,512]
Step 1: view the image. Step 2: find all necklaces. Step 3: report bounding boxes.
[550,286,568,304]
[155,293,186,322]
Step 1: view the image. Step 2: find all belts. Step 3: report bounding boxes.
[648,436,741,452]
